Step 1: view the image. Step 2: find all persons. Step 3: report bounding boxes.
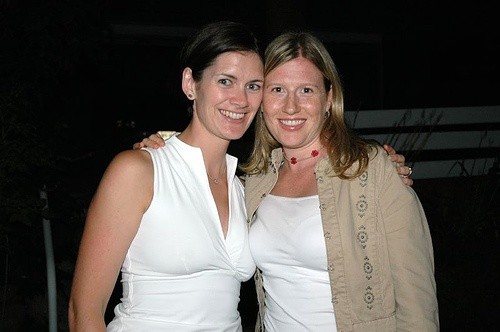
[133,33,441,332]
[68,22,415,332]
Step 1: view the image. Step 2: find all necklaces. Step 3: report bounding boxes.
[207,165,226,184]
[282,146,324,164]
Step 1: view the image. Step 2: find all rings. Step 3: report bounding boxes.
[402,165,413,179]
